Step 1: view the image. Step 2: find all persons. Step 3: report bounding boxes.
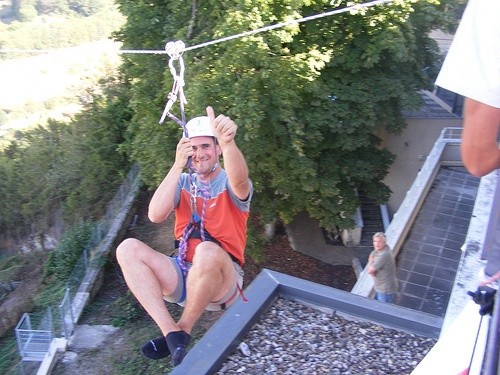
[368,232,399,305]
[434,0,500,178]
[116,105,254,365]
[481,271,500,287]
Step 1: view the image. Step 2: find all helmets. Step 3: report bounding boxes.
[182,116,217,139]
